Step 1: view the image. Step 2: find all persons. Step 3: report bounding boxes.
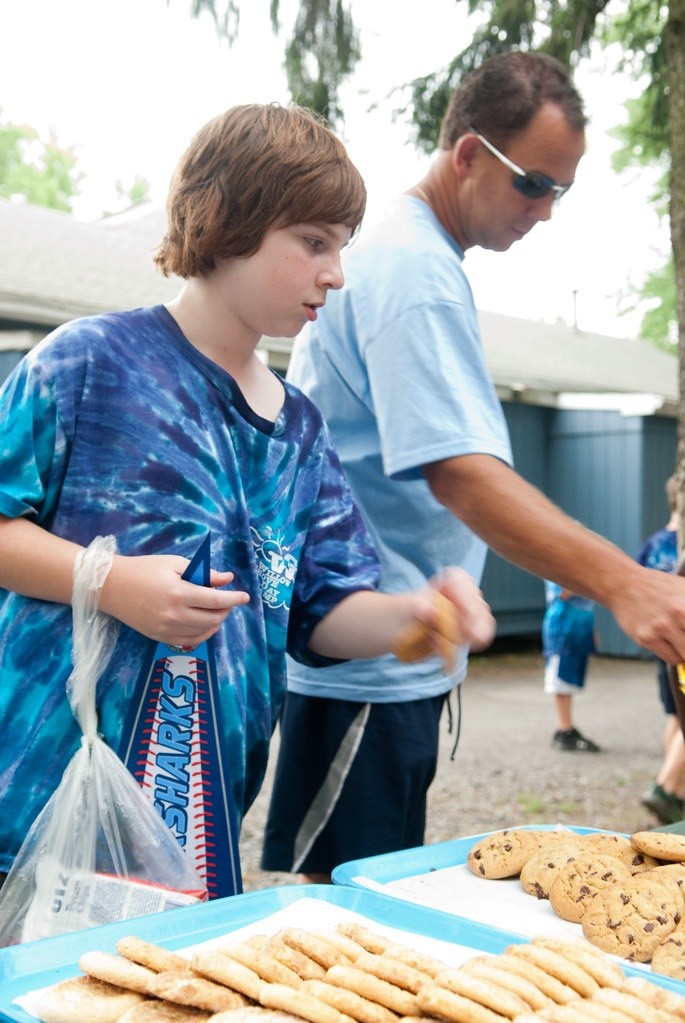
[541,578,600,753]
[259,50,685,885]
[638,474,685,825]
[1,105,498,940]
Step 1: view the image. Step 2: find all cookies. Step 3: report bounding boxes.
[36,932,685,1023]
[467,826,685,985]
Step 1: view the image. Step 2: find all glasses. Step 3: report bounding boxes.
[468,126,575,200]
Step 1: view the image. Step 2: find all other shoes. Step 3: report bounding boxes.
[640,782,685,823]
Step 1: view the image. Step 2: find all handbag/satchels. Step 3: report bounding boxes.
[0,535,204,948]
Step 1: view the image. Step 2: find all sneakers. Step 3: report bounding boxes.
[553,728,598,751]
